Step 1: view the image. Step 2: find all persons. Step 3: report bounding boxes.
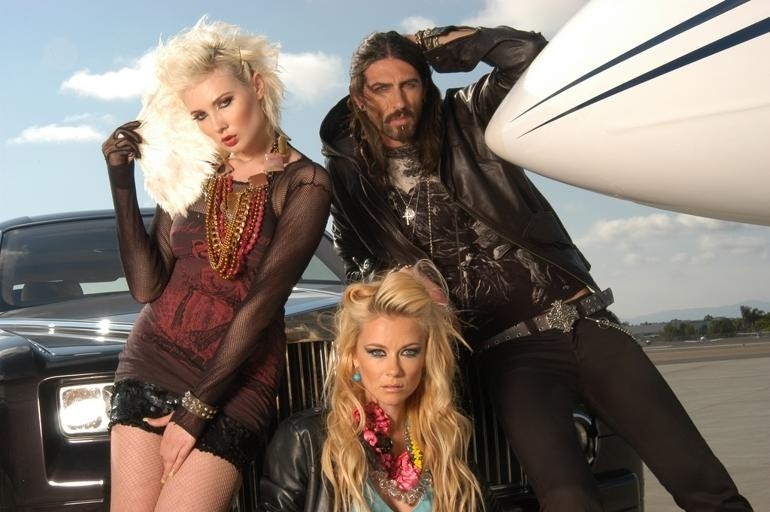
[319,25,754,512]
[258,258,486,511]
[101,14,333,512]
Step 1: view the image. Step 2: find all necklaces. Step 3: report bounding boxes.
[383,153,421,227]
[202,131,286,281]
[357,406,432,507]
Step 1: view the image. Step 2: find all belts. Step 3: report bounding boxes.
[473,287,614,355]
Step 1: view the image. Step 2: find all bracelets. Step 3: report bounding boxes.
[180,389,219,422]
[414,27,438,56]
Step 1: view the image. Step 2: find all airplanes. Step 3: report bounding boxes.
[683,334,725,347]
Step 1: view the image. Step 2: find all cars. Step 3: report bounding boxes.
[0,207,646,512]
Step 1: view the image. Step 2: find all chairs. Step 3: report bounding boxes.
[20,278,83,303]
